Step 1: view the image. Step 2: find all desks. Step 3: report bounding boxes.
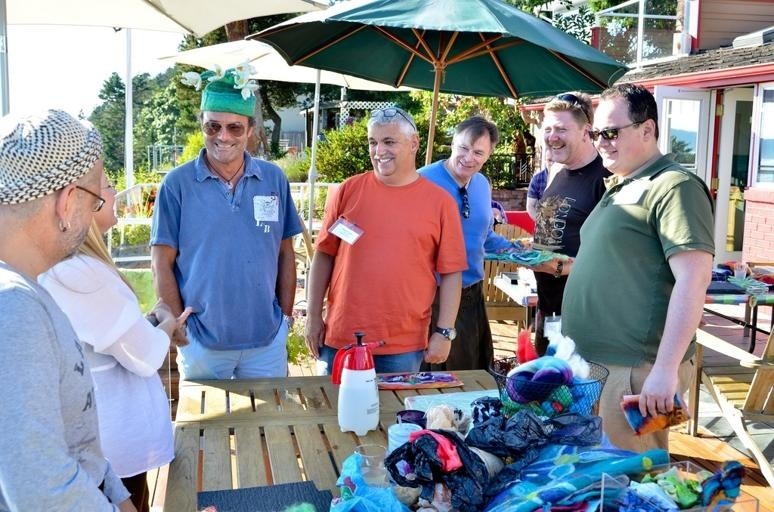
[707,265,774,354]
[162,369,734,512]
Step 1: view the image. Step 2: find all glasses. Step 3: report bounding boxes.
[367,106,417,133]
[585,120,643,142]
[457,184,473,221]
[200,119,250,139]
[552,90,592,128]
[66,176,106,216]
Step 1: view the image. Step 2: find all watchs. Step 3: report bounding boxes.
[434,324,458,341]
[283,314,295,328]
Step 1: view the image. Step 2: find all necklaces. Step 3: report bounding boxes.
[205,155,246,190]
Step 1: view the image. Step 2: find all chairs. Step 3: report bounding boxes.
[292,216,314,263]
[687,325,774,487]
[483,211,536,332]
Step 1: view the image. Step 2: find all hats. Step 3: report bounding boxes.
[198,67,259,120]
[0,97,105,210]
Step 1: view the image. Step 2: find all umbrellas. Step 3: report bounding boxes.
[243,0,629,165]
[158,38,410,298]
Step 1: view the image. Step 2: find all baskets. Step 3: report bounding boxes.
[485,350,611,433]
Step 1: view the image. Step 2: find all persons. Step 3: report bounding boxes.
[305,106,469,375]
[415,116,512,371]
[525,149,555,223]
[36,168,194,512]
[150,63,302,380]
[525,82,718,453]
[1,105,141,512]
[526,89,613,356]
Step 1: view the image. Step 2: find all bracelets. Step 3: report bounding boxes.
[554,261,565,280]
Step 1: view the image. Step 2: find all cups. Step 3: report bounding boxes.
[355,442,388,490]
[734,262,748,280]
